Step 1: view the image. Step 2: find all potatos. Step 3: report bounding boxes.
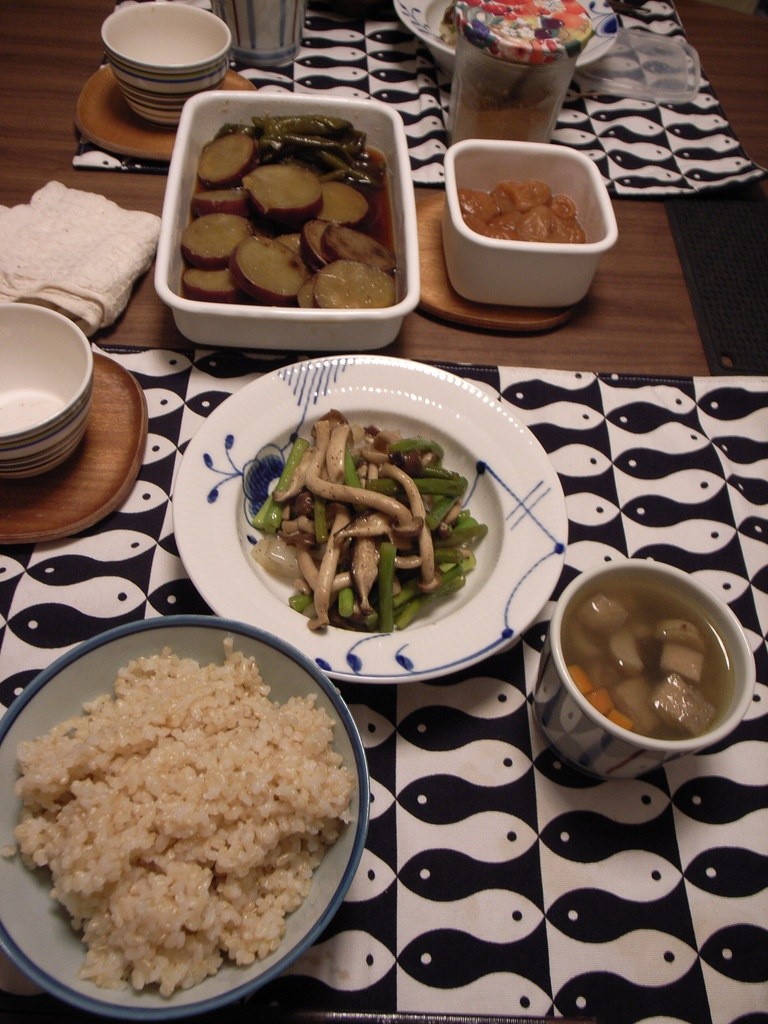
[178,130,396,311]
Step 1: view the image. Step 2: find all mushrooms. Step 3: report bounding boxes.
[272,408,464,634]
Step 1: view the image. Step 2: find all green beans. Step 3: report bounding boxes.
[215,113,378,185]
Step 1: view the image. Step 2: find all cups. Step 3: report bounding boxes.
[450,0,591,146]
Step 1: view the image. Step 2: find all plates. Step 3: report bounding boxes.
[172,354,570,681]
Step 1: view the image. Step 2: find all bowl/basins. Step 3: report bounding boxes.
[100,2,232,125]
[532,561,754,778]
[154,91,421,349]
[0,303,95,480]
[394,1,620,82]
[444,140,619,307]
[1,616,368,1021]
[212,0,305,69]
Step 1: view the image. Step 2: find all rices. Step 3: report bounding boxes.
[0,635,355,1000]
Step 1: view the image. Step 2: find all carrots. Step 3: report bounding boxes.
[575,678,633,731]
